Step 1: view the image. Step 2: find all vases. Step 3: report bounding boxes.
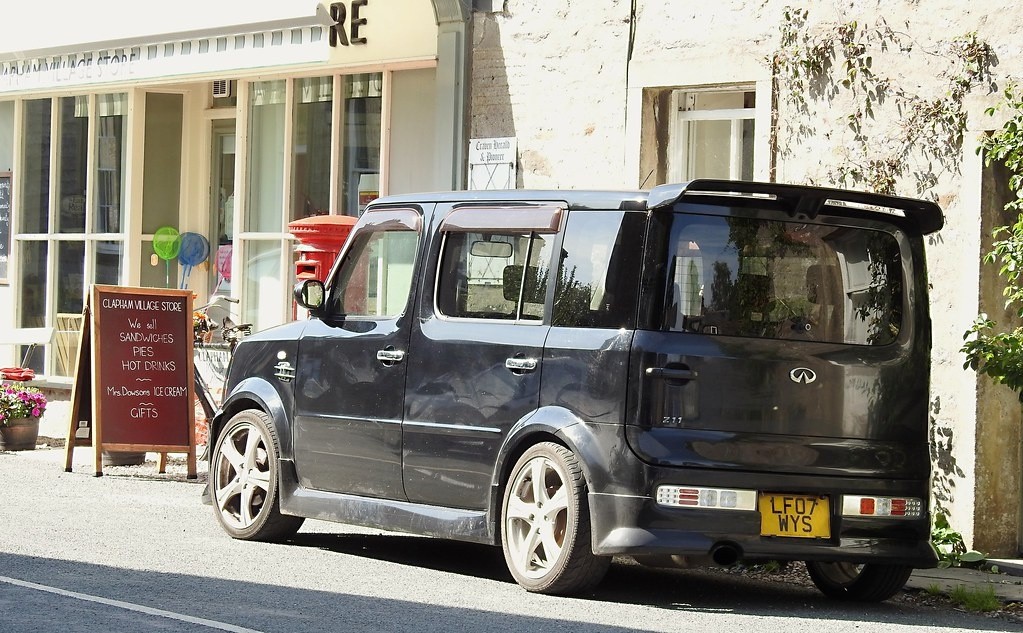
[0,415,39,450]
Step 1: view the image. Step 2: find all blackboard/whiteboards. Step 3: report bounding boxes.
[0,172,13,285]
[65,283,197,452]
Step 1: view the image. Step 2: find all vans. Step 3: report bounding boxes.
[207,177,943,608]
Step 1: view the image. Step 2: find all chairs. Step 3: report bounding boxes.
[728,273,777,340]
[804,265,843,341]
[502,265,548,320]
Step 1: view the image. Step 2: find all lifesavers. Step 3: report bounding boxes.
[0,367,35,381]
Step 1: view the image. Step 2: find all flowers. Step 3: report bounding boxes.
[0,381,47,426]
